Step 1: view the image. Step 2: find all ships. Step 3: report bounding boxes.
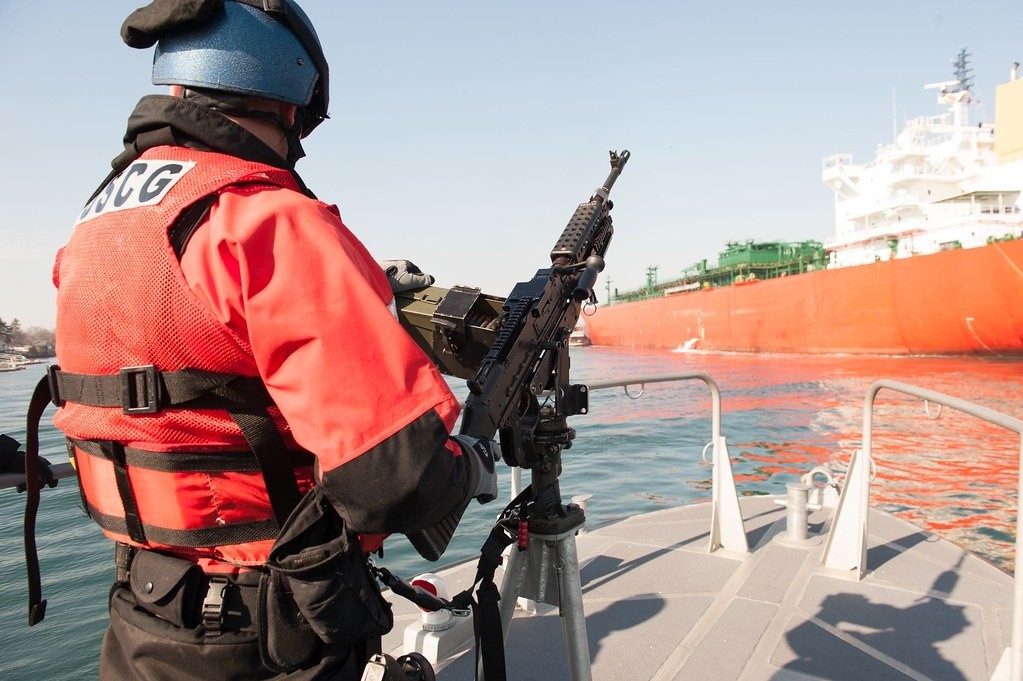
[580,42,1023,359]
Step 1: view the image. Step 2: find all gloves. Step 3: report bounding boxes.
[453,434,502,505]
[378,260,435,293]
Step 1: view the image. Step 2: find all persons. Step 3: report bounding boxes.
[54,0,500,681]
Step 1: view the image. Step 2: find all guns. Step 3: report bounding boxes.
[395,150,632,562]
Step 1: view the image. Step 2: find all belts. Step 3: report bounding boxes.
[114,541,259,633]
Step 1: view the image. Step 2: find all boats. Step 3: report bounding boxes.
[570,330,590,347]
[0,353,43,364]
[0,357,26,372]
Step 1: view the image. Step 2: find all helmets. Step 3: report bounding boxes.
[152,0,330,139]
